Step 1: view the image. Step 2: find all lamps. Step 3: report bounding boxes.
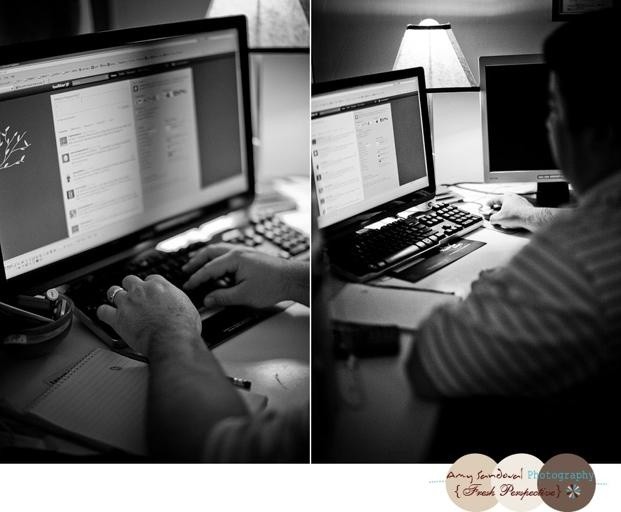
[200,1,310,210]
[393,18,481,206]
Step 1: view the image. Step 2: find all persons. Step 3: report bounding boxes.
[97,242,311,464]
[402,9,621,463]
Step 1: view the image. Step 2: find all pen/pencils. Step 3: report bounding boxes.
[370,283,455,295]
[120,352,251,389]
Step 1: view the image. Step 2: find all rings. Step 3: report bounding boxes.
[109,287,124,304]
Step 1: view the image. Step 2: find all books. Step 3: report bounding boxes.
[24,347,269,460]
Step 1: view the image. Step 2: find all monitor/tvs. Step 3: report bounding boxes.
[0,16,257,300]
[478,53,578,209]
[311,66,436,241]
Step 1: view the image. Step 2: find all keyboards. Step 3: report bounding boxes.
[63,210,310,360]
[329,203,483,283]
